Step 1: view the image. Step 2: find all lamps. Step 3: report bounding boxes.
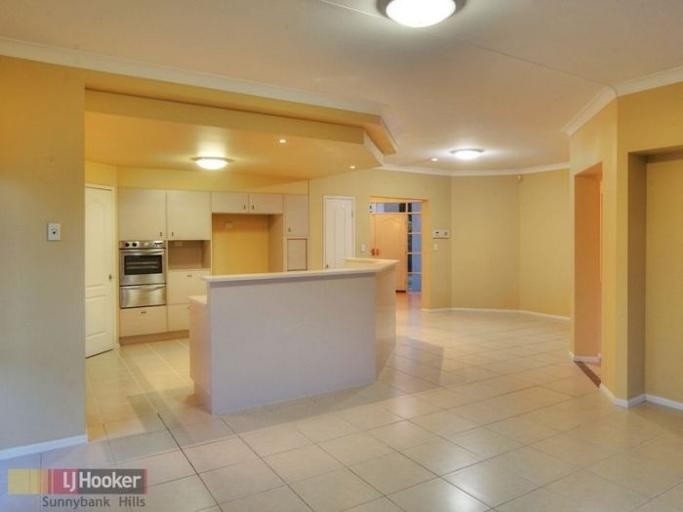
[191,156,233,172]
[449,148,483,160]
[376,1,469,30]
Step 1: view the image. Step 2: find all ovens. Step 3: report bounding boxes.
[119,240,167,308]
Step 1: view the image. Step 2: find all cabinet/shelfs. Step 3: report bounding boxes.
[168,267,209,334]
[118,187,212,243]
[118,304,166,347]
[208,189,282,215]
[269,198,310,272]
